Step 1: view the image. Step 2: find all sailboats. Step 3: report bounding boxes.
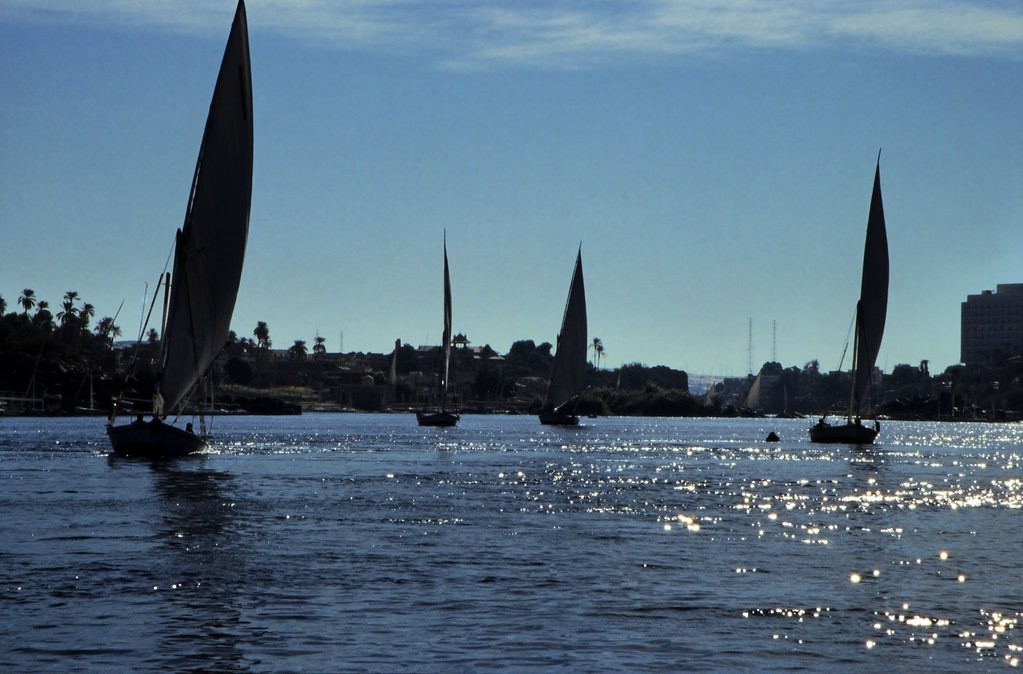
[807,146,891,443]
[415,228,461,429]
[383,359,522,415]
[538,238,593,429]
[103,0,252,463]
[4,338,46,416]
[73,366,132,415]
[339,374,355,413]
